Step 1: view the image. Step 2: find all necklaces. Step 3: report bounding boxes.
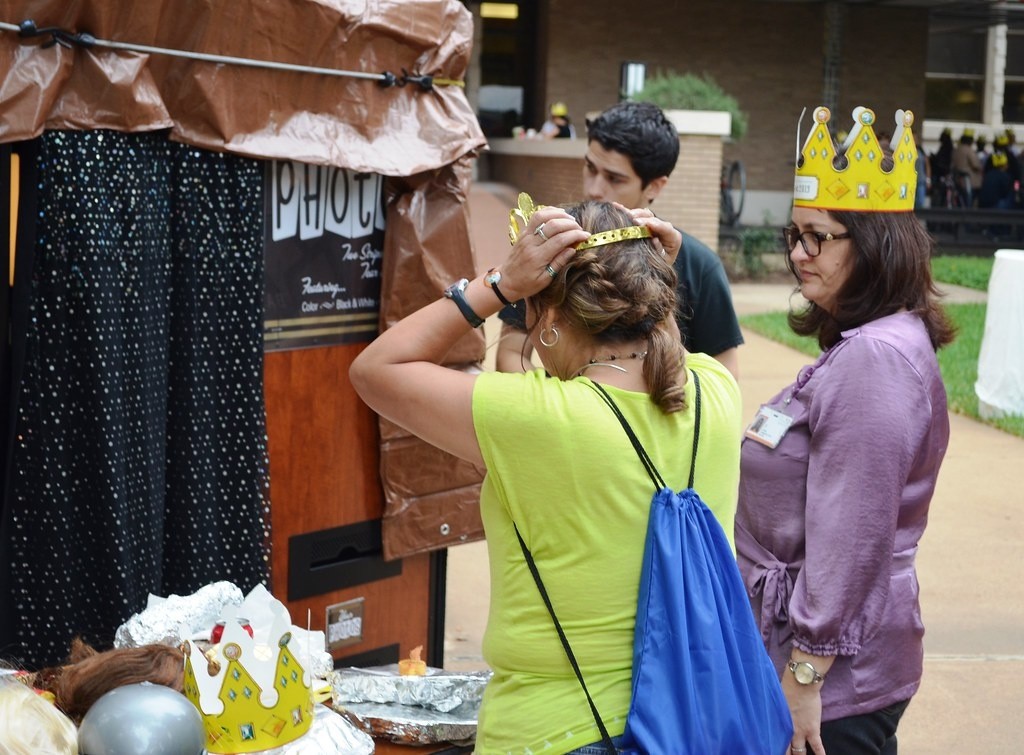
[578,350,650,376]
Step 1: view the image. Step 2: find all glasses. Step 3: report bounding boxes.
[782,226,853,257]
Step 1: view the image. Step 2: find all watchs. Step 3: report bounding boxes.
[443,278,486,329]
[788,658,825,685]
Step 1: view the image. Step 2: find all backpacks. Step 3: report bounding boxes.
[512,378,795,755]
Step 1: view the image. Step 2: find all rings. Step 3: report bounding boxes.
[791,747,807,753]
[545,265,556,277]
[534,222,548,240]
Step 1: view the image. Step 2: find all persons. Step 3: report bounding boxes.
[494,98,745,387]
[534,100,575,140]
[870,126,1024,212]
[349,193,744,755]
[732,134,958,755]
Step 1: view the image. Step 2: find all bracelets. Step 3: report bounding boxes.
[483,266,515,308]
